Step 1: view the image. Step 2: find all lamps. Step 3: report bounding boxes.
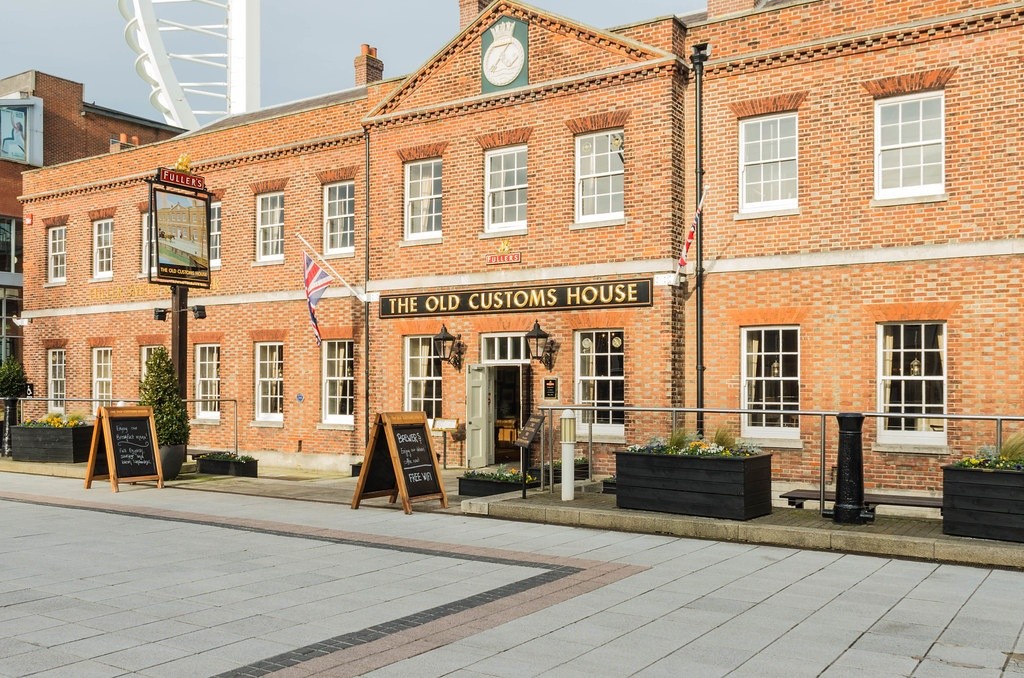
[433,323,463,374]
[346,341,352,377]
[772,329,780,378]
[909,323,921,376]
[278,344,283,377]
[525,319,555,373]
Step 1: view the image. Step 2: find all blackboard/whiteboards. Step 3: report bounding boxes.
[360,413,400,500]
[102,406,163,483]
[86,406,110,481]
[381,410,450,503]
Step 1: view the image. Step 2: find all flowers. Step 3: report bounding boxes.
[20,413,86,428]
[626,434,762,458]
[463,465,537,484]
[957,453,1018,470]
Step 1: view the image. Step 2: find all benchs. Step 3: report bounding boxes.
[780,489,944,517]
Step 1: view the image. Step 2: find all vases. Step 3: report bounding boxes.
[611,451,774,522]
[10,425,95,463]
[940,465,1024,544]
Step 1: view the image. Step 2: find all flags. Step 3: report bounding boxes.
[304,252,334,347]
[678,199,703,268]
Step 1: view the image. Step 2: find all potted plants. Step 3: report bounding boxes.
[194,452,258,477]
[138,347,191,480]
